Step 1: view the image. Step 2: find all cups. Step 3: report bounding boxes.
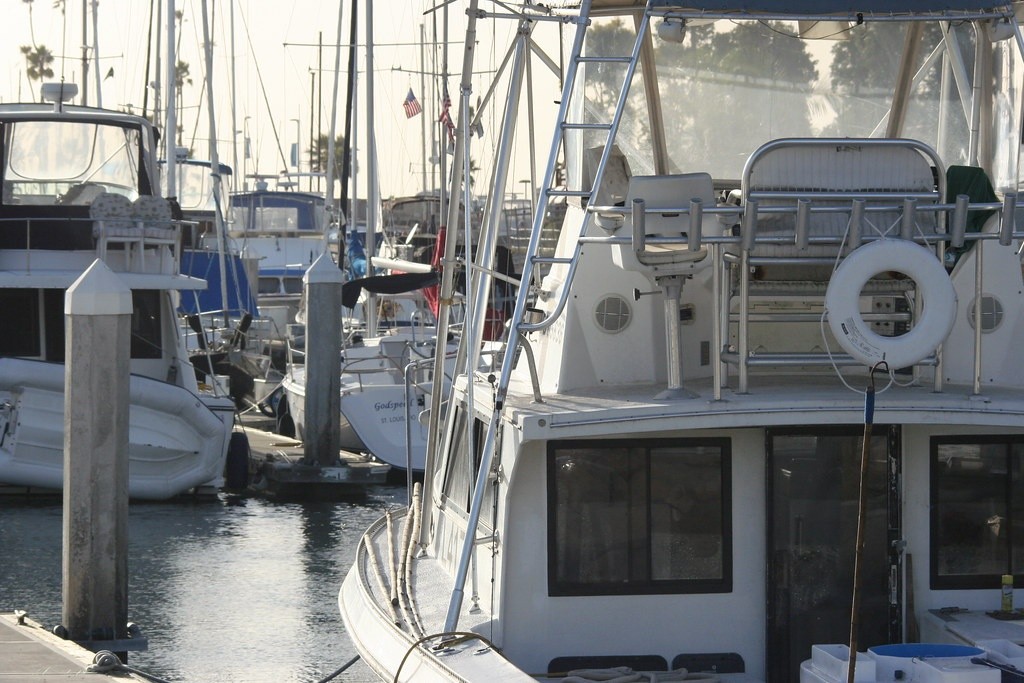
[1002,575,1013,612]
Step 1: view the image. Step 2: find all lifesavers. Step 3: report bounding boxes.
[825,238,958,371]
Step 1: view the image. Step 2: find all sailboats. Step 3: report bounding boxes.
[0,1,1024,682]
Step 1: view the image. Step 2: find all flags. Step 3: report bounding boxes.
[402,87,423,118]
